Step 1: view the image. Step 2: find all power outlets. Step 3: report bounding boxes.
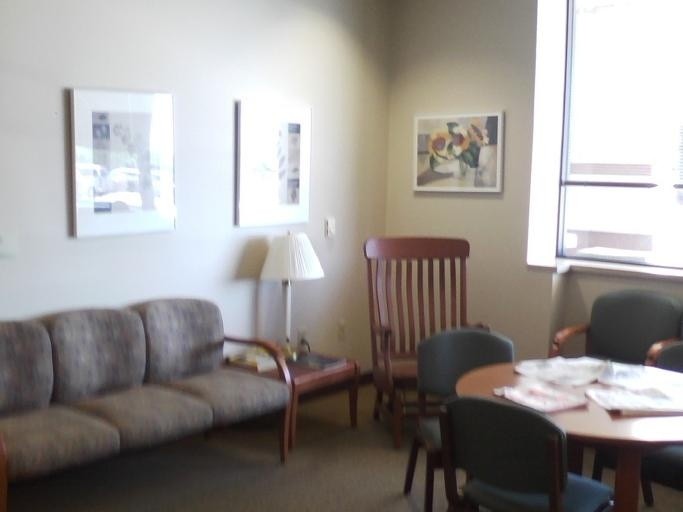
[297,330,310,349]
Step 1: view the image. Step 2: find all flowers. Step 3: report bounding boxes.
[428,122,487,176]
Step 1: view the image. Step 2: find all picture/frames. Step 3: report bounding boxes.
[64,85,177,240]
[232,97,313,232]
[412,112,506,199]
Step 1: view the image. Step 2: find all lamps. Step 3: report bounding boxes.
[259,232,327,359]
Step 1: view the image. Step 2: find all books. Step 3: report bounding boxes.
[294,353,348,370]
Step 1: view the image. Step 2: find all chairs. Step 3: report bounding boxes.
[364,233,493,447]
[0,297,292,511]
[437,393,618,510]
[396,326,518,510]
[546,289,683,510]
[642,334,683,511]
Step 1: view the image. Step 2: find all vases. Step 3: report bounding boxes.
[433,154,467,177]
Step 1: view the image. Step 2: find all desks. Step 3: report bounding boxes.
[451,357,682,510]
[220,350,362,451]
